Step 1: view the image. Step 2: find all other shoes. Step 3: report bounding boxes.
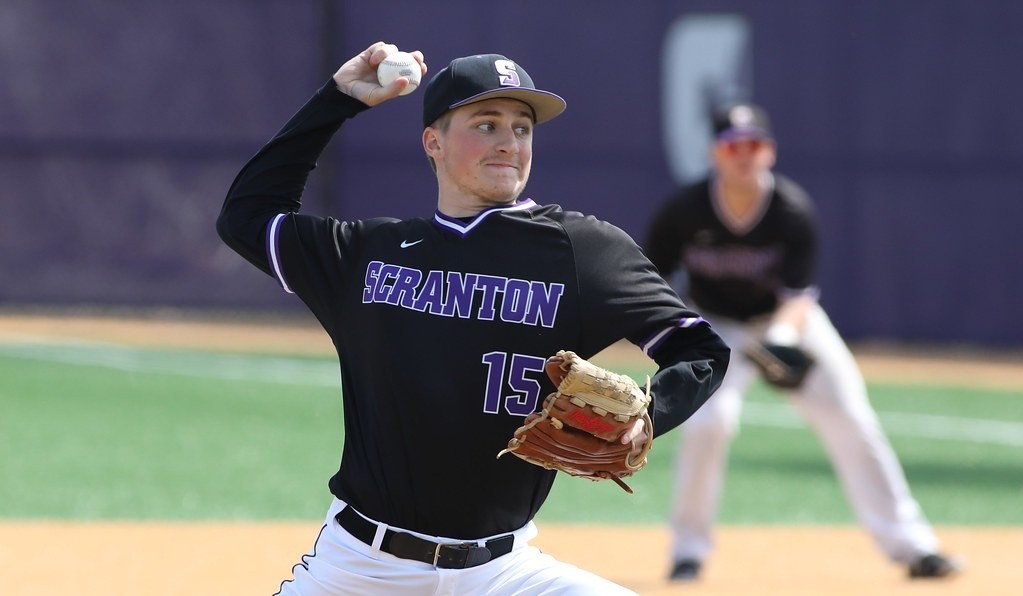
[910,553,958,577]
[667,563,697,583]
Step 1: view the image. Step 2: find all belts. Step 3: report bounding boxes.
[335,504,514,570]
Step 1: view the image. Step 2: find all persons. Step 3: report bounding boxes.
[215,41,731,596]
[643,106,965,580]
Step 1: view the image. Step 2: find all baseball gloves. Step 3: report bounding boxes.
[506,348,655,483]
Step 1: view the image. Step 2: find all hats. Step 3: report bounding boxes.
[711,102,775,143]
[424,54,567,131]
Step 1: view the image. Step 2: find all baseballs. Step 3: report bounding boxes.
[376,51,422,96]
